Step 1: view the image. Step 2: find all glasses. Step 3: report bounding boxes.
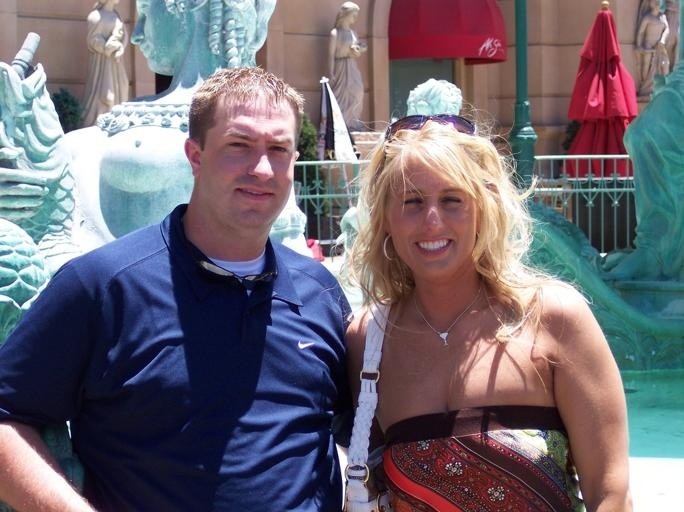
[383,114,474,160]
[181,221,278,289]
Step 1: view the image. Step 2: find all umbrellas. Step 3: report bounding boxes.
[318,74,359,207]
[562,2,639,179]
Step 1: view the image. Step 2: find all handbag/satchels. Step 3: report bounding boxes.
[339,296,389,512]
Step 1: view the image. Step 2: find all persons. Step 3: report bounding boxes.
[317,114,633,512]
[0,66,356,511]
[78,2,131,123]
[407,79,462,119]
[93,0,309,253]
[635,0,669,99]
[327,1,367,130]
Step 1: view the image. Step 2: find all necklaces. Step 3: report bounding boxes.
[409,280,484,347]
